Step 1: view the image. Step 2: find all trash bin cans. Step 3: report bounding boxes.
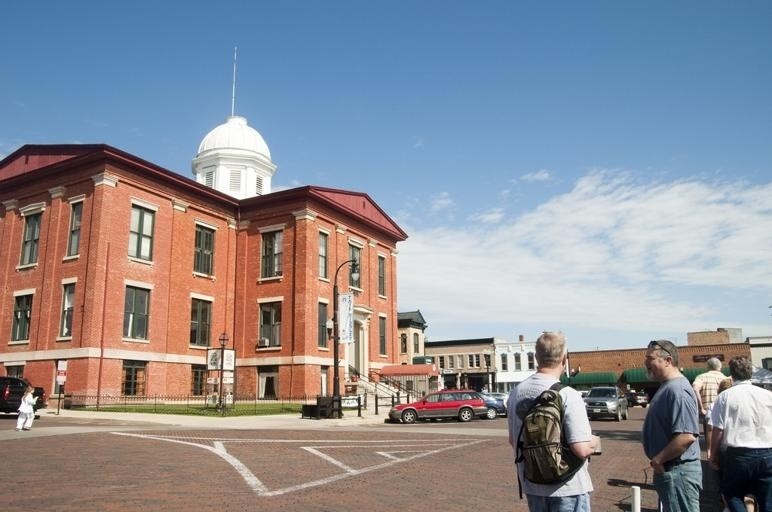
[64,395,72,409]
[303,397,333,420]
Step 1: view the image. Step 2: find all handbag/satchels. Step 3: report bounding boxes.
[698,457,726,512]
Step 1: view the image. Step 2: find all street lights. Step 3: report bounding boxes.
[216,332,231,412]
[331,255,359,417]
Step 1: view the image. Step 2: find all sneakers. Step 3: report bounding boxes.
[14,427,32,433]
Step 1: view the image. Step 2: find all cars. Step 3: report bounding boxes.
[388,387,648,424]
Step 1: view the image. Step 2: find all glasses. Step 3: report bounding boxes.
[648,341,672,357]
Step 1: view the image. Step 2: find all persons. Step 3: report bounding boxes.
[692,355,727,433]
[709,354,772,512]
[506,330,596,512]
[640,338,703,512]
[705,378,731,457]
[16,385,40,431]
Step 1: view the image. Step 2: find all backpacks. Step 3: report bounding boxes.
[515,381,588,486]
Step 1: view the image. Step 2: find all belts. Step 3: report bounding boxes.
[662,457,699,470]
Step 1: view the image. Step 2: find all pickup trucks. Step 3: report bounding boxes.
[0,375,47,418]
